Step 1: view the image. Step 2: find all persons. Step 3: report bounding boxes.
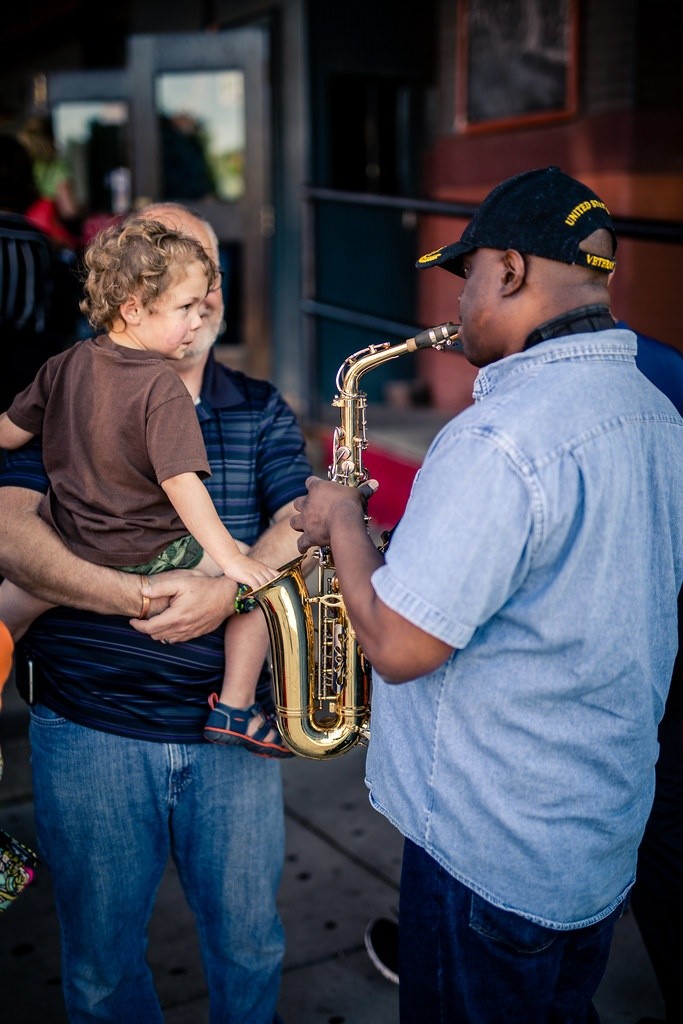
[0,220,291,758]
[290,169,683,1024]
[0,105,230,403]
[0,198,316,1024]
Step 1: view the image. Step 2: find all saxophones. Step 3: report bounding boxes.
[235,318,464,760]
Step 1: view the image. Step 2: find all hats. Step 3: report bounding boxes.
[415,164,618,279]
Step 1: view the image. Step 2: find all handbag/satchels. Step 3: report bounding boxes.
[0,828,41,918]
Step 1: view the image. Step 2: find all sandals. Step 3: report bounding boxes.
[202,692,297,759]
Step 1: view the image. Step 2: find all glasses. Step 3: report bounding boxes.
[209,271,225,291]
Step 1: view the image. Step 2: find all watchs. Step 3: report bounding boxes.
[235,581,256,615]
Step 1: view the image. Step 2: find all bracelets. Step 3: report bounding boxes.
[140,575,149,620]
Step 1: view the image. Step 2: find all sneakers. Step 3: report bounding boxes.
[363,915,400,985]
